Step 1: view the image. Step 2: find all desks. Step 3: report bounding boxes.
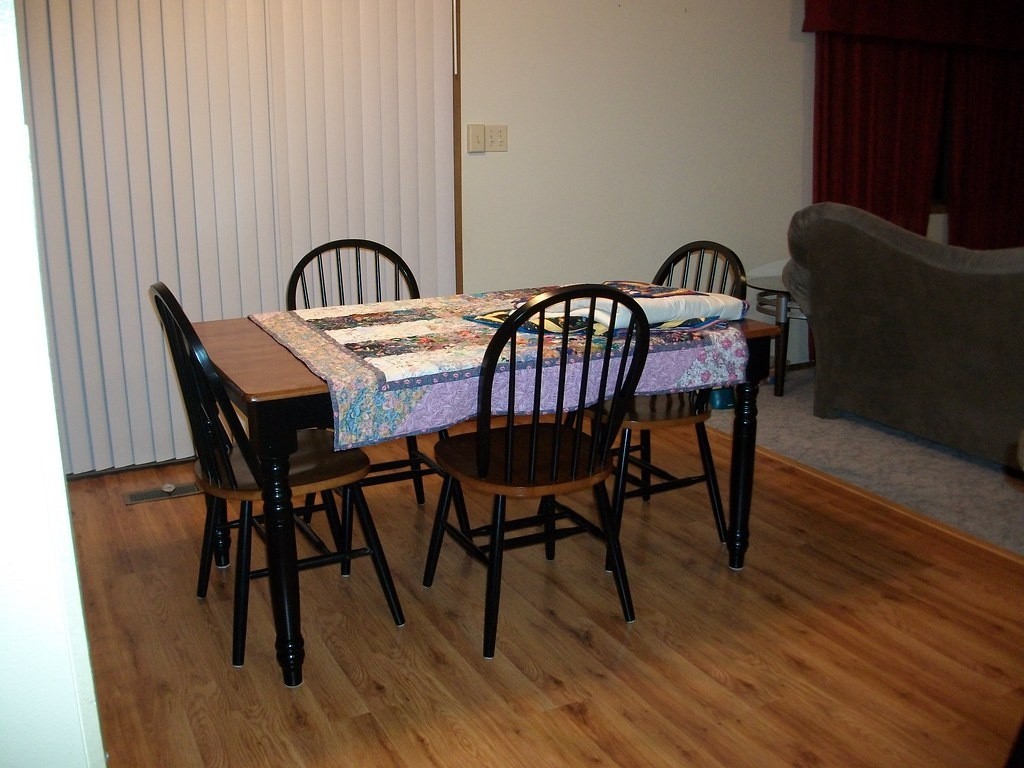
[746,257,814,396]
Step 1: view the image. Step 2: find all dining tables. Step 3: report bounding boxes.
[191,279,780,687]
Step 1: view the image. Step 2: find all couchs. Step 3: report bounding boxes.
[783,201,1024,474]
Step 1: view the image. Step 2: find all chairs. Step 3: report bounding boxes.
[535,239,748,571]
[421,283,651,659]
[148,281,409,668]
[285,239,474,587]
